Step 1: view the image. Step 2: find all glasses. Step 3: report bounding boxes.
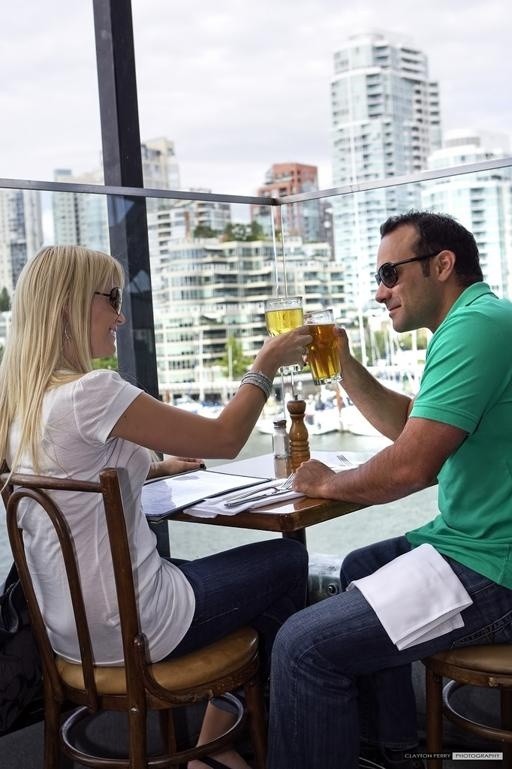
[374,252,440,288]
[95,287,122,316]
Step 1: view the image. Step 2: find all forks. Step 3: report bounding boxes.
[224,471,296,502]
[336,454,356,471]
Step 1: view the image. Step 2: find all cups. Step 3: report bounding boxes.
[262,294,344,388]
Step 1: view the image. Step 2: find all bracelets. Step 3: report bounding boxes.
[240,371,273,402]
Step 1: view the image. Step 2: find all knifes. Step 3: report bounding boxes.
[223,489,293,507]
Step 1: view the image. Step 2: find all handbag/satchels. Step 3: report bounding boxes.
[0,563,44,734]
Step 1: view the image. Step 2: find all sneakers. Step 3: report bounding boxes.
[358,739,425,768]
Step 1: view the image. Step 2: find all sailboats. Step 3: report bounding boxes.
[174,304,437,441]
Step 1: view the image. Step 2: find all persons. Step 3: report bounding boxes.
[268,209,512,769]
[0,245,314,769]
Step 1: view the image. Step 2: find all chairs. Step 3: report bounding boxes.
[421,644,512,769]
[1,458,266,769]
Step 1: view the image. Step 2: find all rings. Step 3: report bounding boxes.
[303,347,306,354]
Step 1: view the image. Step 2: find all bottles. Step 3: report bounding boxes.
[271,418,291,461]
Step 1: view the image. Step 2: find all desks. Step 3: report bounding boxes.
[166,451,438,549]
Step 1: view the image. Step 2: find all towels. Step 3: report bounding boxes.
[345,542,473,651]
